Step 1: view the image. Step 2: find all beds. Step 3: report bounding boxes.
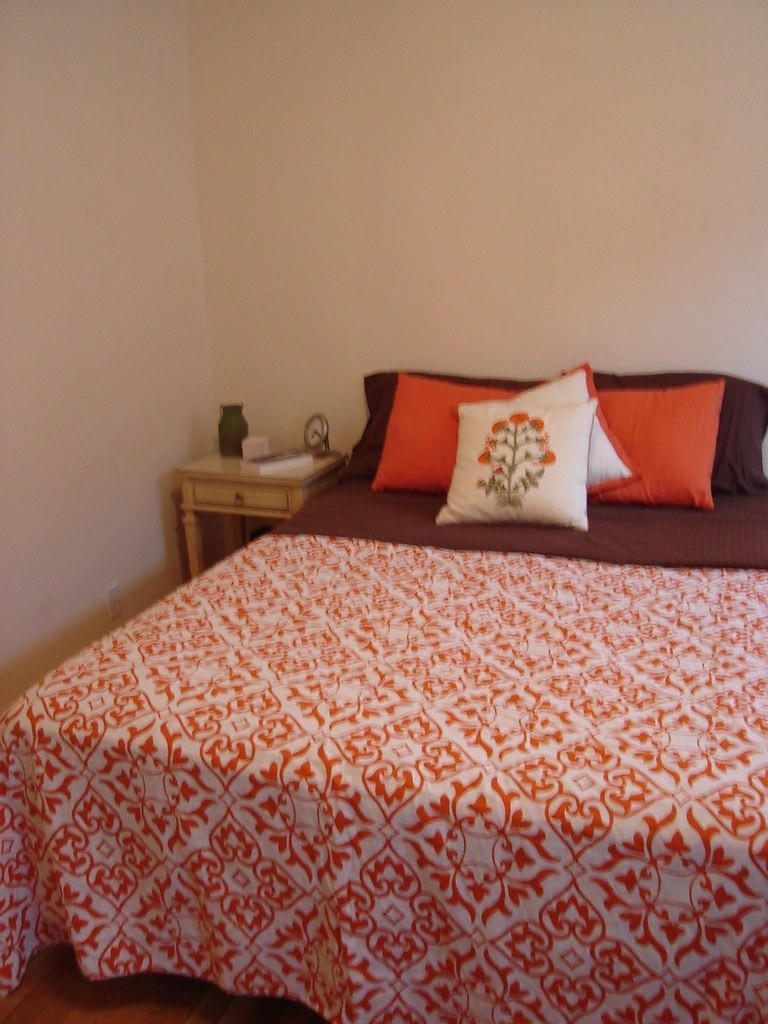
[0,475,768,1024]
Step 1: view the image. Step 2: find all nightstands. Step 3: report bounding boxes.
[175,451,349,581]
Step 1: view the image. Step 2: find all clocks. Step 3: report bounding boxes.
[303,414,331,456]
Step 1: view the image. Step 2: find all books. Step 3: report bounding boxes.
[248,448,313,475]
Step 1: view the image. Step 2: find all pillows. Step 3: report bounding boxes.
[593,372,768,497]
[514,364,641,496]
[337,371,550,486]
[435,398,598,532]
[373,374,725,510]
